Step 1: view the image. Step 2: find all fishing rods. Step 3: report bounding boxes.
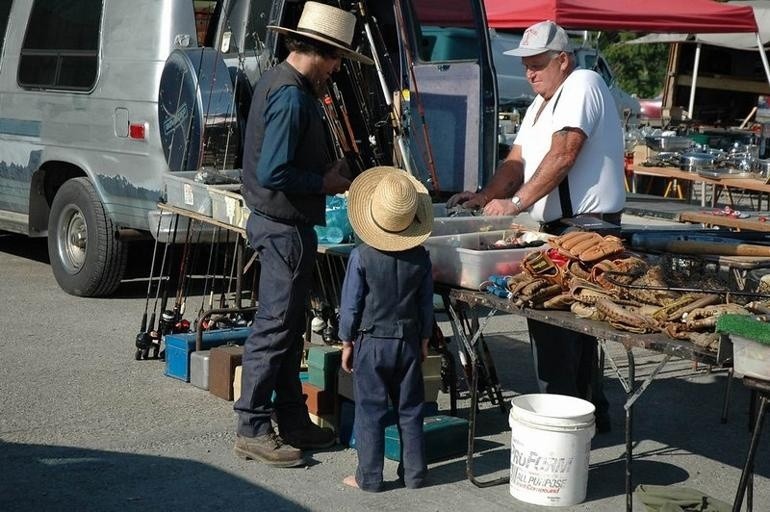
[136,1,284,360]
[143,1,214,358]
[305,1,504,412]
[137,70,186,361]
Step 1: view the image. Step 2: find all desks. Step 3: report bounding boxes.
[624,119,769,242]
[153,201,770,512]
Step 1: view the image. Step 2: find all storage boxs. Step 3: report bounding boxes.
[209,183,251,230]
[163,169,244,218]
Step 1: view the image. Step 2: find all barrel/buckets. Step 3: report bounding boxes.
[508,393,596,506]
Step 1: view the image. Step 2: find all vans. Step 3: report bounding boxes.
[420,27,642,127]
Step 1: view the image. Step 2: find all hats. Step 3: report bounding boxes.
[269,1,376,66]
[348,164,434,253]
[503,19,574,57]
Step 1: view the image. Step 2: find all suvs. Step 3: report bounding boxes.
[0,0,501,299]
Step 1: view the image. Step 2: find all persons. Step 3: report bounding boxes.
[444,20,626,434]
[338,164,434,494]
[232,0,375,468]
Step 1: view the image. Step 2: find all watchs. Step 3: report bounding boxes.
[511,195,523,213]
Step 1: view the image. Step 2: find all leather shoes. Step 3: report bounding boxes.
[278,421,336,448]
[234,432,309,467]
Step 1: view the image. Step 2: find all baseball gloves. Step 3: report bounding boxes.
[480,231,770,352]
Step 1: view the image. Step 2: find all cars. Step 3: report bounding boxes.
[637,90,664,119]
